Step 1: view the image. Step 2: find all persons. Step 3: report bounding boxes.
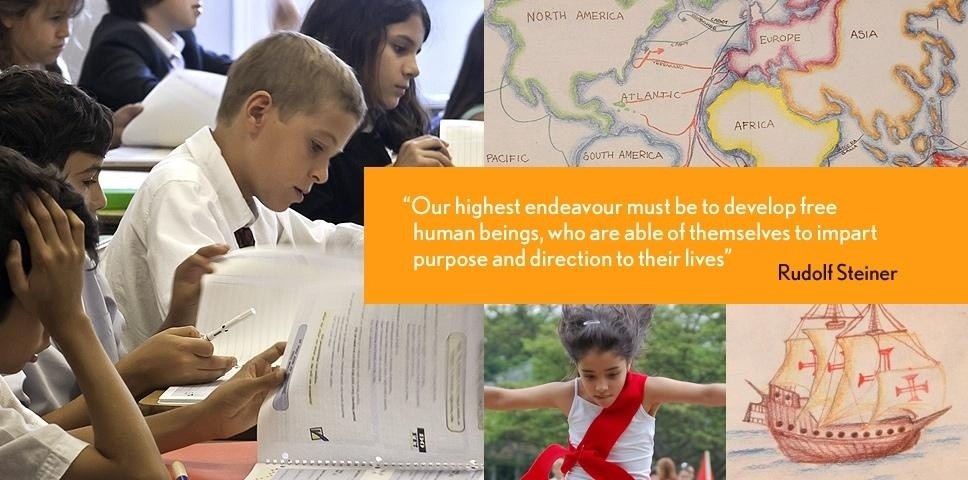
[483,304,726,480]
[0,0,484,480]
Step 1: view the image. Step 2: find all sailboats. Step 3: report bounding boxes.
[737,303,951,469]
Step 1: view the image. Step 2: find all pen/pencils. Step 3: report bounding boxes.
[201,308,257,341]
[432,146,442,151]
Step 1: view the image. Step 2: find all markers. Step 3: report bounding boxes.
[172,461,189,480]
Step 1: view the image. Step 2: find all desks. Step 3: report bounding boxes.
[137,381,226,417]
[97,208,126,237]
